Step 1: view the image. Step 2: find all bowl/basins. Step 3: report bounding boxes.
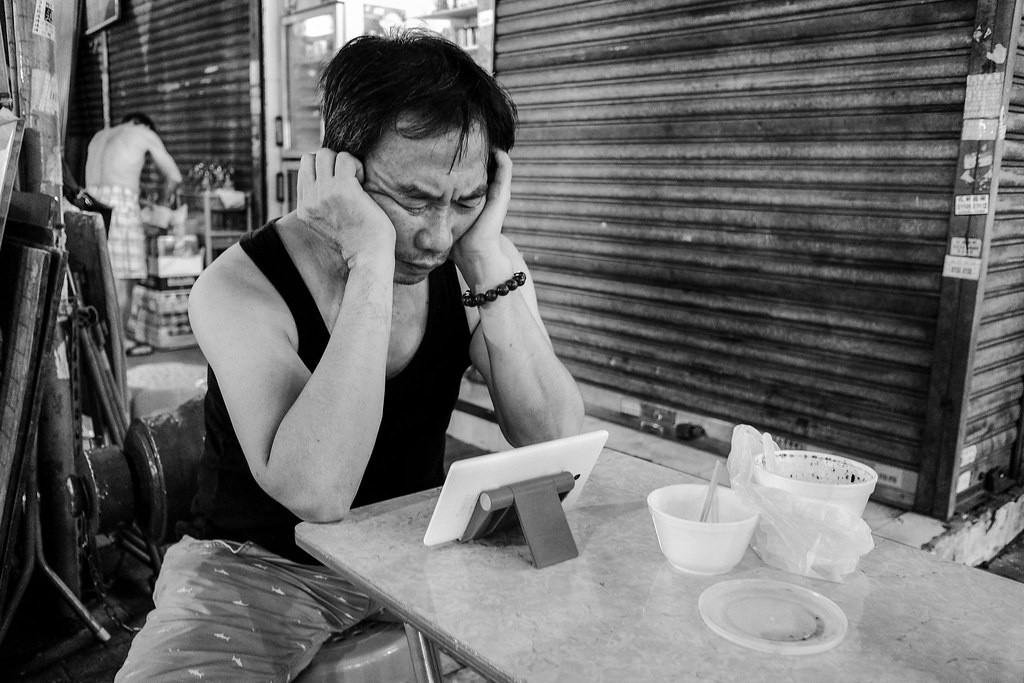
[751,452,878,565]
[647,485,760,575]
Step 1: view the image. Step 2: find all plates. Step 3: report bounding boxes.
[697,579,848,655]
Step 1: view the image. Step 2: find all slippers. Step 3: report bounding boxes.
[125,337,153,357]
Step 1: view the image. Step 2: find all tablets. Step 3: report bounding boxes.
[423,430,609,546]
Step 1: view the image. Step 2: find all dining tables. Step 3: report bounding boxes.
[293,446,1024,683]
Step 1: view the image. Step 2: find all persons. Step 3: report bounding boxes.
[85,111,183,357]
[114,27,584,683]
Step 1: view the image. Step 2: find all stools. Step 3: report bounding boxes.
[290,620,419,683]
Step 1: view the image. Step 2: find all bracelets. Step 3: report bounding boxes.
[462,272,526,308]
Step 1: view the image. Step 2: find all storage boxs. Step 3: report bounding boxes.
[125,233,205,351]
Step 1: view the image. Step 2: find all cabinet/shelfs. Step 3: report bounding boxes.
[412,1,479,53]
[177,185,252,271]
